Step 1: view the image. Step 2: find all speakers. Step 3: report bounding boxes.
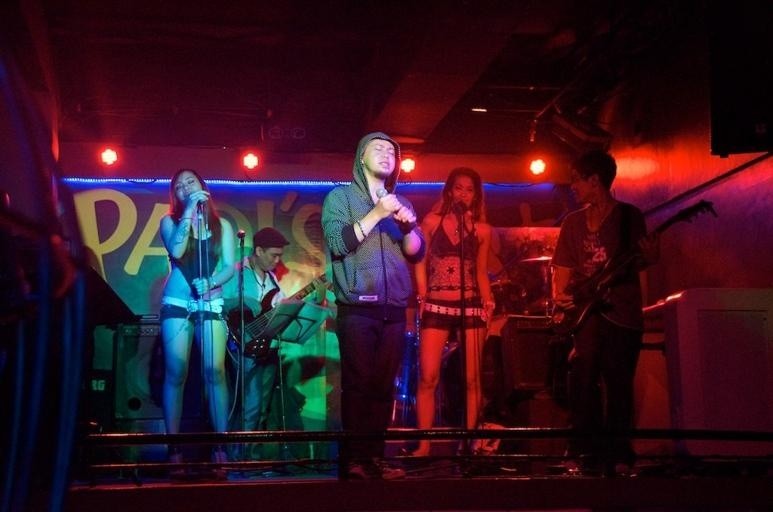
[111,324,165,420]
[704,0,773,158]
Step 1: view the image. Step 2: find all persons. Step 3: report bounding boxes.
[321,131,425,482]
[158,167,235,480]
[543,150,660,479]
[408,165,497,458]
[219,225,326,473]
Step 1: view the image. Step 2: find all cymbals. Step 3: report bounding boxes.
[522,256,552,261]
[525,298,550,313]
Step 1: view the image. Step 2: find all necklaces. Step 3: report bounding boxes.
[450,211,470,235]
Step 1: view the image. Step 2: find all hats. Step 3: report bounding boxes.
[253,227,290,247]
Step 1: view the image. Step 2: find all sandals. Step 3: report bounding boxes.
[210,444,229,481]
[163,446,190,482]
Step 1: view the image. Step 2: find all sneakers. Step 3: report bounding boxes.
[373,458,406,480]
[347,462,369,479]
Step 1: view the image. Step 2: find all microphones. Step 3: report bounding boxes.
[376,188,400,215]
[237,229,245,238]
[188,192,207,204]
[455,201,468,215]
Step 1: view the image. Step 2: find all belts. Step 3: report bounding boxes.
[160,295,224,314]
[423,303,483,317]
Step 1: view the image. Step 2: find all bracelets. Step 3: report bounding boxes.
[209,275,216,290]
[178,217,194,221]
[355,220,368,241]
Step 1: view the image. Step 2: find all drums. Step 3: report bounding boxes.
[491,279,519,315]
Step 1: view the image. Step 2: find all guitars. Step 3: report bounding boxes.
[226,273,329,358]
[547,200,718,334]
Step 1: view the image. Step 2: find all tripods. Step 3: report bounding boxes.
[272,343,307,475]
